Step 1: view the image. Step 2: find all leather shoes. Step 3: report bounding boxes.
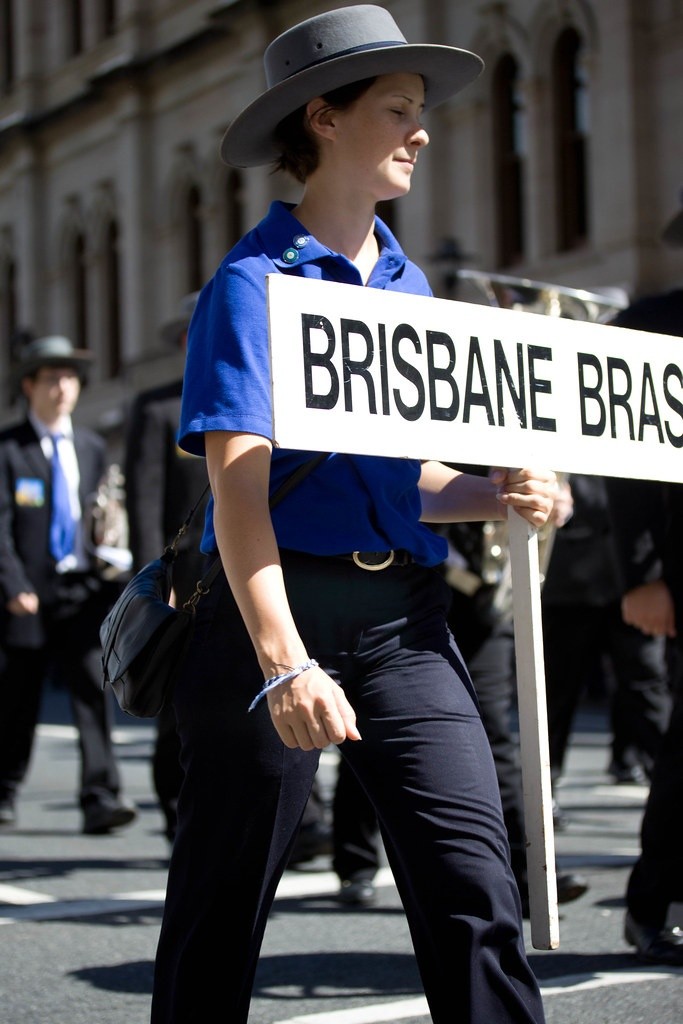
[556,874,587,904]
[629,929,683,967]
[341,879,376,906]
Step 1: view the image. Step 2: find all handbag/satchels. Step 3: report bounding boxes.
[94,551,196,720]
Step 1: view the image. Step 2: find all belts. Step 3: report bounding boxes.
[287,546,417,572]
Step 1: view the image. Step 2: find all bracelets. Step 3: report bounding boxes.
[247,659,318,713]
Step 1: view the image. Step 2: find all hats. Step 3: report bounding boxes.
[219,6,486,168]
[13,335,97,380]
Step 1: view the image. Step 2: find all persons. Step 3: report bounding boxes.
[158,200,683,967]
[0,335,139,834]
[148,5,559,1024]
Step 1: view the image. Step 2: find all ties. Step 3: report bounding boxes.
[47,432,78,562]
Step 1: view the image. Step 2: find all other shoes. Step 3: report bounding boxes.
[289,817,332,868]
[0,789,13,828]
[79,798,134,835]
[607,761,651,786]
